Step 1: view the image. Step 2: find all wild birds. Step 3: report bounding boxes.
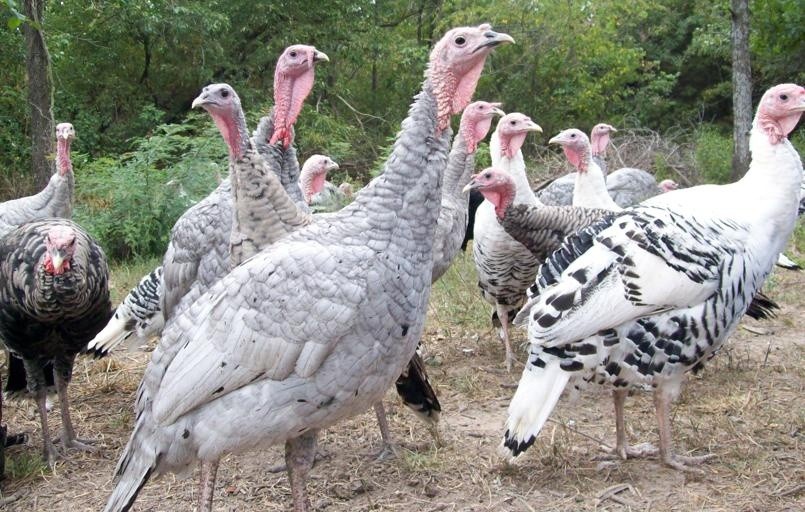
[463,84,805,471]
[0,121,76,240]
[87,25,514,512]
[0,216,118,480]
